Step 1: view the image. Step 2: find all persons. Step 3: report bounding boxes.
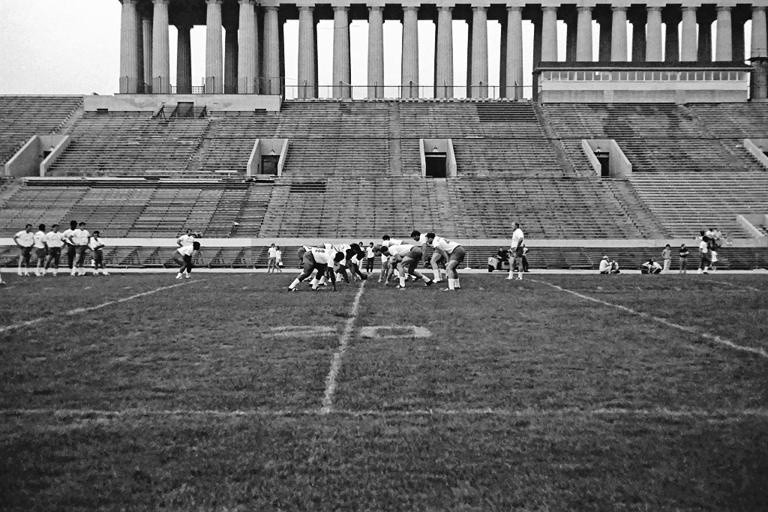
[497,221,529,281]
[661,244,673,274]
[266,230,466,293]
[696,228,724,274]
[609,258,620,274]
[640,258,662,274]
[173,229,203,280]
[678,244,690,275]
[13,220,110,277]
[598,255,613,274]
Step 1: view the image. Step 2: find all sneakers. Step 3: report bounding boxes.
[424,279,433,287]
[311,286,322,291]
[288,287,300,291]
[303,277,313,282]
[318,282,328,286]
[395,285,407,291]
[433,277,462,293]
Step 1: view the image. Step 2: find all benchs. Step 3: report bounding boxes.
[1,94,768,272]
